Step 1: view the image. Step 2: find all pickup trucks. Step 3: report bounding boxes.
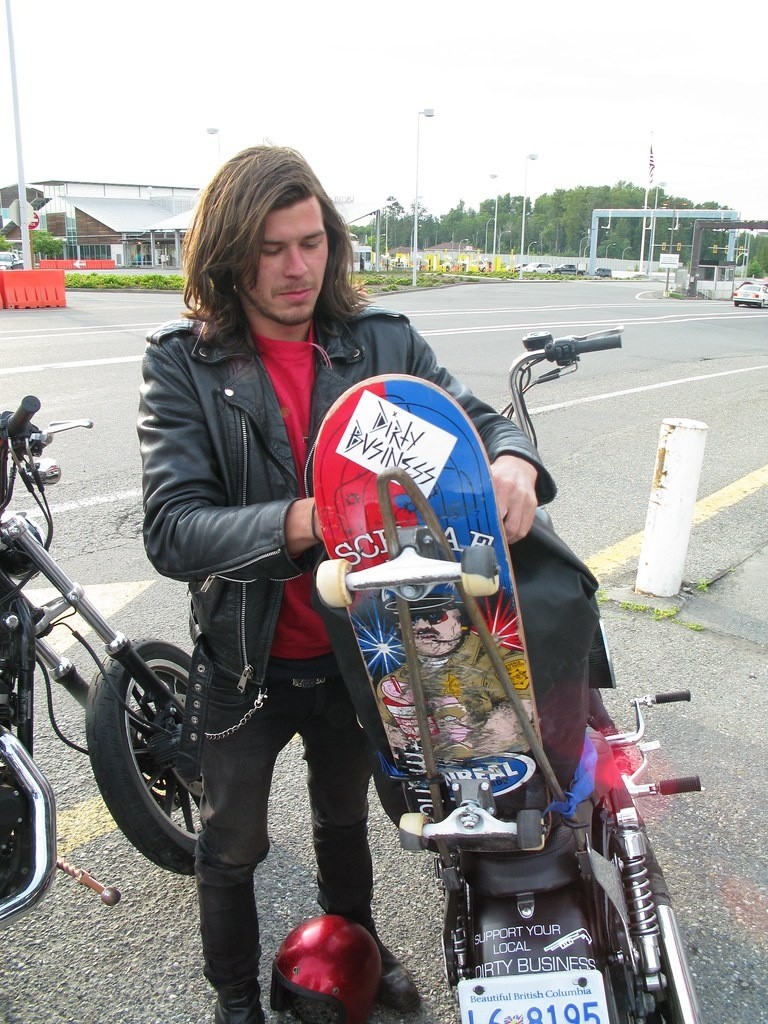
[553,263,586,276]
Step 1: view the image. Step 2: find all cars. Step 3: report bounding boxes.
[512,262,529,272]
[596,268,612,279]
[0,250,24,271]
[732,280,768,308]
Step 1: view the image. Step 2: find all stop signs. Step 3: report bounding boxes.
[27,212,40,230]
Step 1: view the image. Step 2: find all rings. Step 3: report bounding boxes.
[512,536,519,541]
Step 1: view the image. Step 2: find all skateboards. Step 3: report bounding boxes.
[310,374,554,859]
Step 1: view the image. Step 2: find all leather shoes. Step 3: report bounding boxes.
[364,919,420,1012]
[215,978,265,1024]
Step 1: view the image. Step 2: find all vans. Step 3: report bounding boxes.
[522,262,553,275]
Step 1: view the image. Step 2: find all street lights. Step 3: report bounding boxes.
[206,127,221,172]
[484,218,496,254]
[459,238,468,253]
[498,230,511,254]
[621,246,632,260]
[527,241,538,255]
[490,172,498,254]
[413,108,435,286]
[410,224,422,262]
[605,244,616,258]
[520,153,538,257]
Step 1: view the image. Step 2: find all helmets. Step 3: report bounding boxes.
[270,914,381,1023]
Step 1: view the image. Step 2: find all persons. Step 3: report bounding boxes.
[138,146,557,1024]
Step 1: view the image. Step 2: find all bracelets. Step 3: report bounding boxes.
[311,502,324,545]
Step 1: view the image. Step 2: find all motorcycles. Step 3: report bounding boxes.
[0,390,216,938]
[396,325,704,1022]
